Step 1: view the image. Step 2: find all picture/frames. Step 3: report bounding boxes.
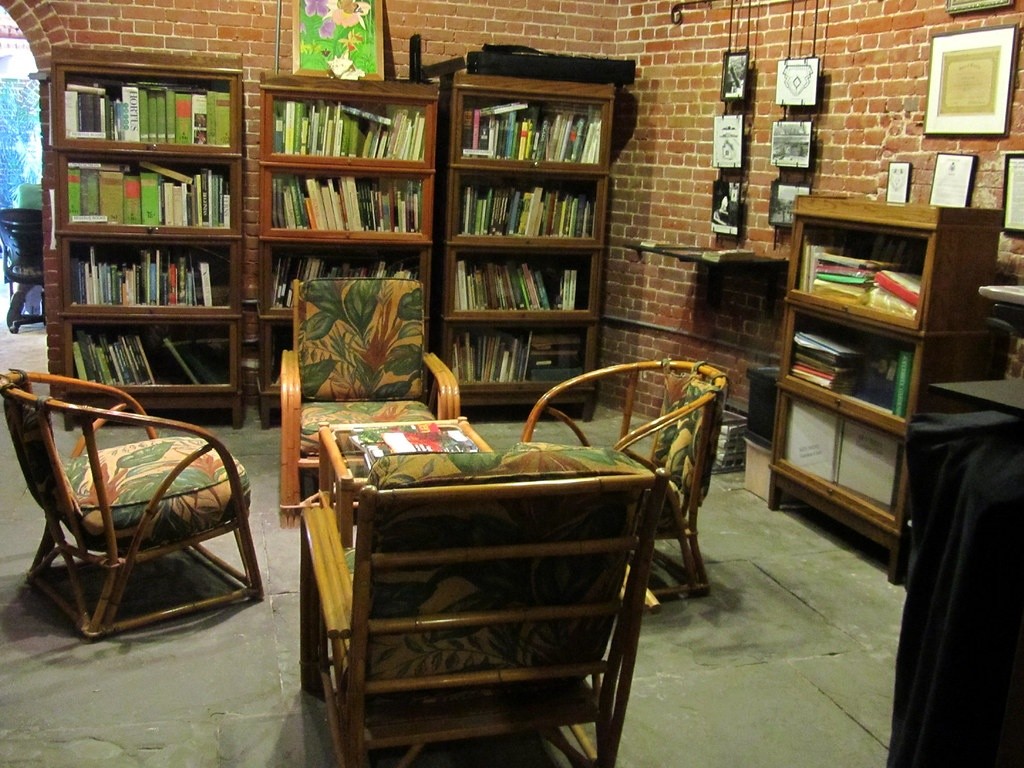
[1001,153,1024,235]
[713,113,745,170]
[776,57,820,107]
[945,0,1015,14]
[886,160,912,205]
[921,21,1020,138]
[292,0,385,81]
[929,151,979,207]
[767,181,813,227]
[720,51,750,101]
[710,181,742,237]
[770,121,814,170]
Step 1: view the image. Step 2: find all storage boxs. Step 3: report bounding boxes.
[840,422,898,507]
[789,401,840,482]
[744,445,805,507]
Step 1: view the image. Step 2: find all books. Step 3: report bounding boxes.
[348,421,481,473]
[72,331,199,384]
[272,175,420,233]
[702,248,756,263]
[461,100,603,164]
[454,258,577,310]
[273,98,424,160]
[272,251,419,307]
[641,240,690,249]
[74,244,215,307]
[799,239,920,321]
[450,329,585,382]
[791,330,910,418]
[64,75,230,148]
[66,161,230,228]
[462,184,595,237]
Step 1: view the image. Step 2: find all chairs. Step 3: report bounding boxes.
[522,358,731,611]
[0,208,46,332]
[299,440,672,768]
[277,278,465,523]
[0,367,265,637]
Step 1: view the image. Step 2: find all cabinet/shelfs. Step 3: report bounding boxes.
[765,196,1004,580]
[254,70,442,431]
[428,69,618,420]
[49,49,249,424]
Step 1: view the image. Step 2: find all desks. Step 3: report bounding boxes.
[627,240,792,269]
[928,376,1024,418]
[978,284,1024,306]
[317,415,494,549]
